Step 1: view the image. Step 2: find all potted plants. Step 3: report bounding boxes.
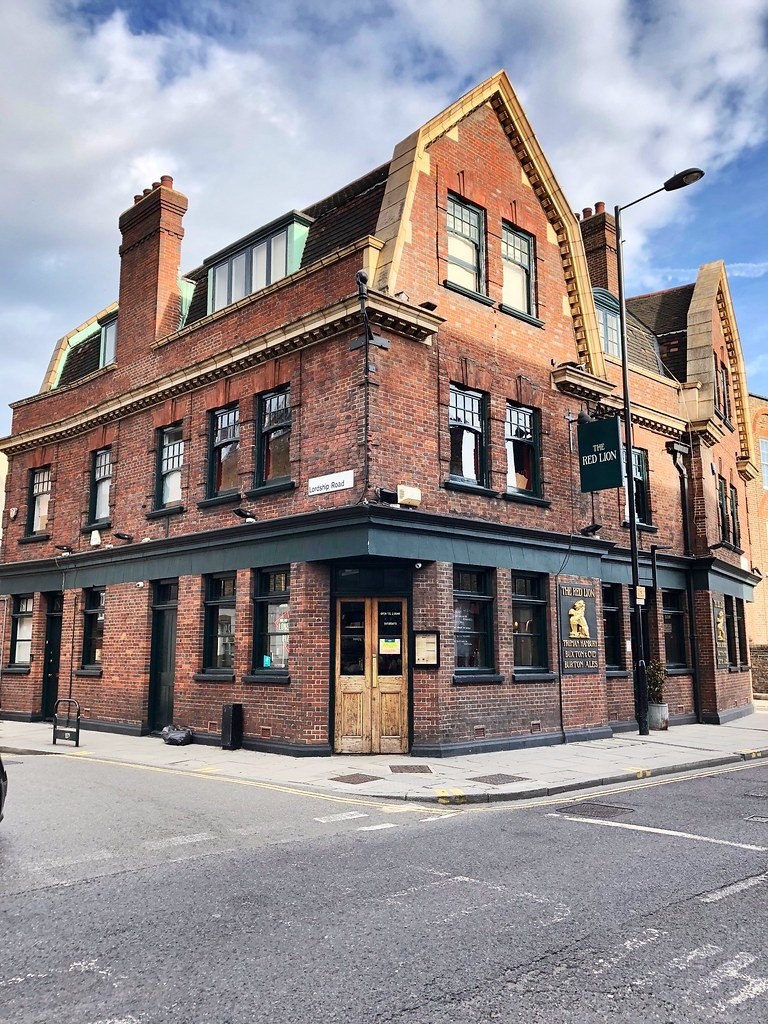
[645,659,669,730]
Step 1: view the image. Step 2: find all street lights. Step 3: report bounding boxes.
[614,164,706,736]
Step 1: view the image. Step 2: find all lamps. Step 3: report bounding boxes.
[232,509,256,519]
[55,544,72,552]
[709,542,726,550]
[581,524,603,537]
[113,532,134,541]
[577,400,595,424]
[380,487,398,505]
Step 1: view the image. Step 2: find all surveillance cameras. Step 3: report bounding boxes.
[415,563,422,568]
[136,582,144,588]
